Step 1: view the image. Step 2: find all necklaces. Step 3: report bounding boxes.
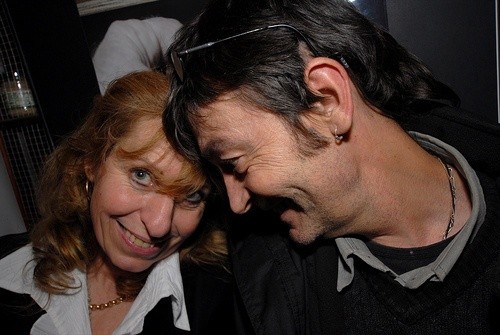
[86,291,129,311]
[439,158,458,242]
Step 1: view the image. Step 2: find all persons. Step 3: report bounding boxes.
[165,1,500,335]
[0,69,249,335]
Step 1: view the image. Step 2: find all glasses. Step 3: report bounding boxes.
[166,22,320,85]
